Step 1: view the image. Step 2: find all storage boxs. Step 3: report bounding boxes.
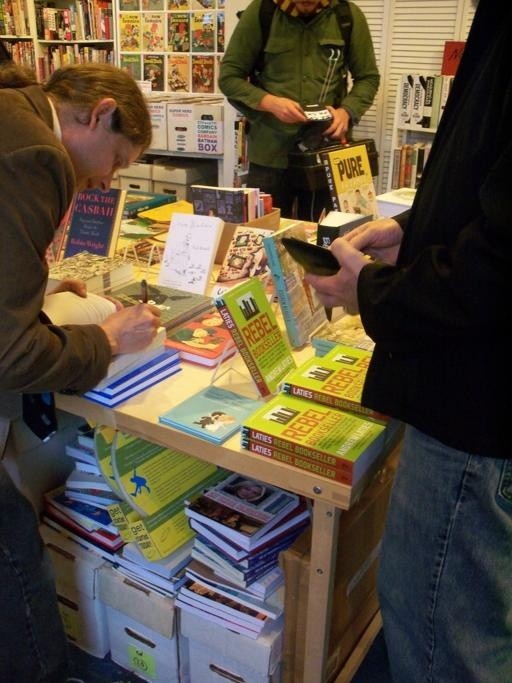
[106,95,229,200]
[38,524,281,683]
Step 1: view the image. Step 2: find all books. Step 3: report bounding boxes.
[0,1,116,84]
[400,71,454,133]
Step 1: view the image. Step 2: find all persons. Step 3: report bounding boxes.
[0,60,162,682]
[217,0,381,220]
[302,0,512,683]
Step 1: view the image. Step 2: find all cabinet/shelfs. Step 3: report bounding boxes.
[45,194,384,682]
[1,0,115,88]
[374,73,437,219]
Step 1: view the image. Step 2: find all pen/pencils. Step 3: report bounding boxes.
[141,279,147,301]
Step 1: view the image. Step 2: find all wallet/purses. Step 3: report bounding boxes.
[279,236,374,276]
